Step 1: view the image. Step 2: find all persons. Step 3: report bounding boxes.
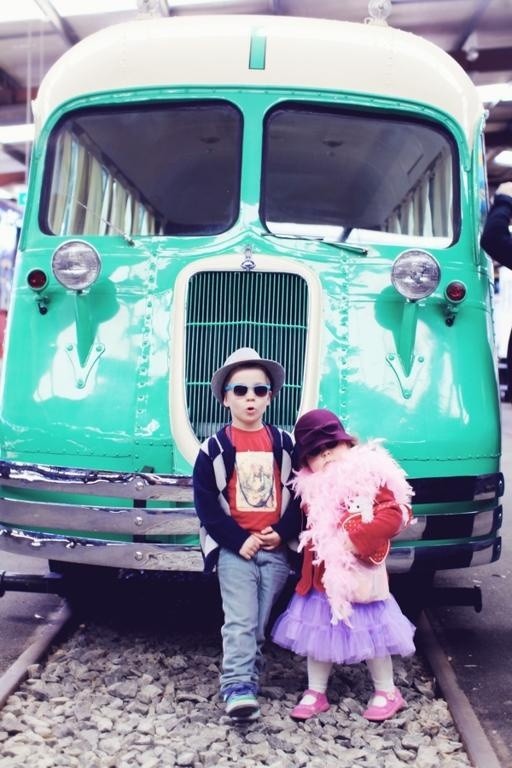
[190,347,295,720]
[480,180,511,273]
[268,409,416,723]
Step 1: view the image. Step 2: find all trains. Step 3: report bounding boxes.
[2,11,512,622]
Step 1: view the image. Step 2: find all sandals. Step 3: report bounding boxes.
[363,688,403,720]
[289,688,330,719]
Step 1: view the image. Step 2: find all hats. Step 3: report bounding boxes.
[211,347,285,410]
[295,409,354,465]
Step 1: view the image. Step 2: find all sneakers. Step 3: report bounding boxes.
[226,689,260,721]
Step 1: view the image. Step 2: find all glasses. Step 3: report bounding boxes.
[225,384,271,397]
[307,441,338,456]
